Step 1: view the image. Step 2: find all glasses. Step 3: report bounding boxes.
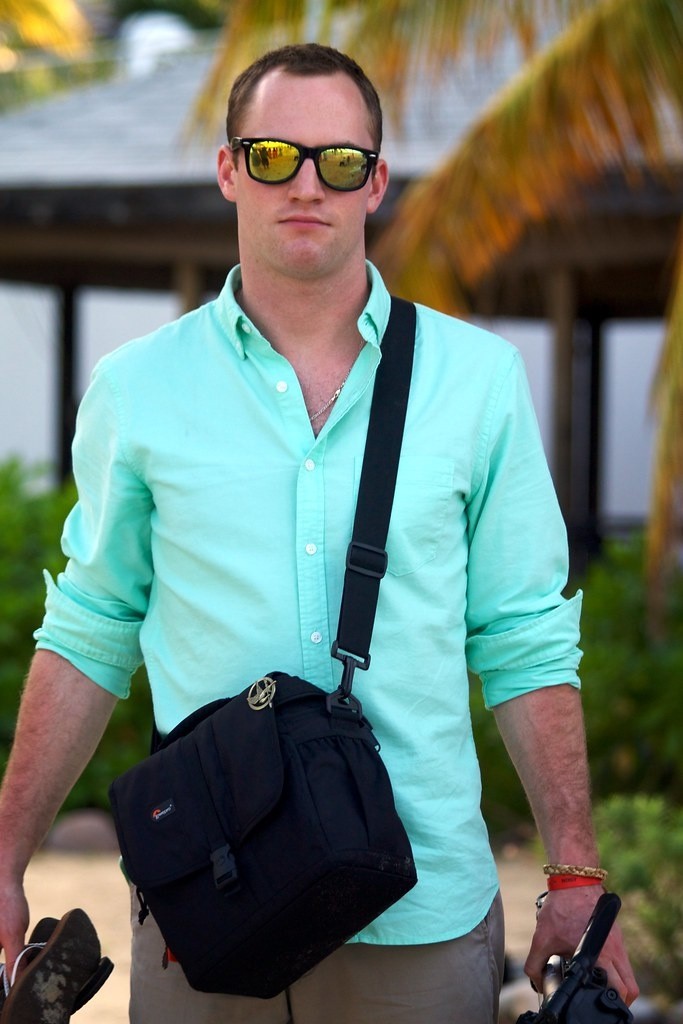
[229,137,378,191]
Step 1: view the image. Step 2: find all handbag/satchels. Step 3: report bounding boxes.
[108,669,415,999]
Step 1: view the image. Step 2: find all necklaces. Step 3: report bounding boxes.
[309,340,365,420]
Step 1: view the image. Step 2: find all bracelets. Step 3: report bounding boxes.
[546,875,603,892]
[543,864,607,880]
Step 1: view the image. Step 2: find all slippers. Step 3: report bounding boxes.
[0,908,114,1024]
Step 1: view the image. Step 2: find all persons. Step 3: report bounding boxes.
[339,155,351,166]
[250,146,282,169]
[1,42,639,1023]
